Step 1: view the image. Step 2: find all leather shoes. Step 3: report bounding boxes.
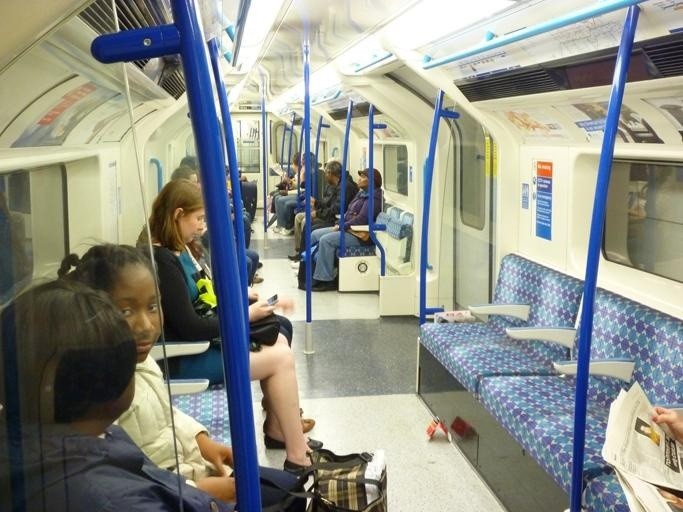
[262,418,324,449]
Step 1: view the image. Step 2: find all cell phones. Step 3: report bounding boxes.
[266,293,279,306]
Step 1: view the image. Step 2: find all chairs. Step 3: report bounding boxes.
[373,206,413,276]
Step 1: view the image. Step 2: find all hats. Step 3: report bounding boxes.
[357,167,382,188]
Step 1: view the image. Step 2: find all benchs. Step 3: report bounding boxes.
[419,254,585,399]
[580,474,629,511]
[335,202,391,293]
[474,286,683,495]
[148,339,232,447]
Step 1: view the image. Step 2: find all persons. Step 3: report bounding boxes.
[651,405,683,444]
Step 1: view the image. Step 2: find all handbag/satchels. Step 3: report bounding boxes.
[307,447,388,512]
[297,245,339,292]
[209,299,281,346]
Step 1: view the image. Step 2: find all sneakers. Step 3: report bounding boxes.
[261,395,304,417]
[283,451,313,479]
[311,280,335,291]
[287,253,303,263]
[272,226,295,235]
[251,262,265,284]
